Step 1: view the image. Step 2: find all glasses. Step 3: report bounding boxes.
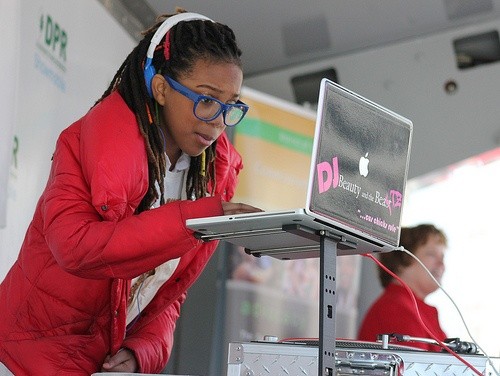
[163,73,249,127]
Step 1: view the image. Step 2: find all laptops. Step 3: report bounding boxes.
[185,77,413,260]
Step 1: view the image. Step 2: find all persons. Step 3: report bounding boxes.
[356,224,448,352]
[0,7,265,376]
